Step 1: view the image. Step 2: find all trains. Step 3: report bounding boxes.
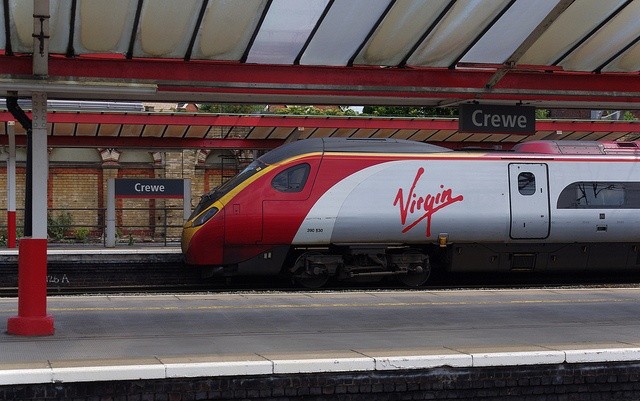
[180,136,640,282]
